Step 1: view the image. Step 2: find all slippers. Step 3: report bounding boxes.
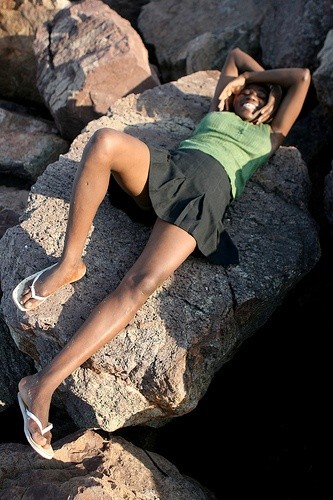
[16,391,55,459]
[12,261,87,311]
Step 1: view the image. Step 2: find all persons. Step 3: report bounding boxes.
[12,47,311,459]
